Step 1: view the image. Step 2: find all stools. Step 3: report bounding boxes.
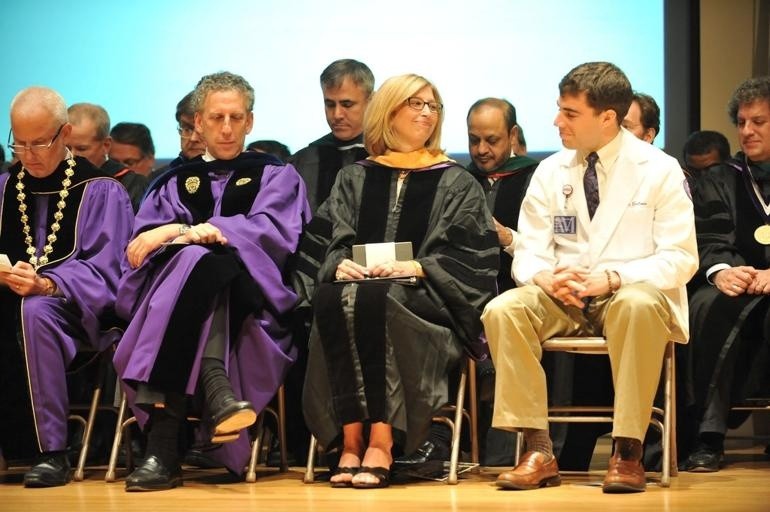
[512,332,683,492]
[59,369,102,483]
[302,343,485,487]
[103,384,290,486]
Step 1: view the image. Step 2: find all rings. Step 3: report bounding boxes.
[758,283,763,288]
[16,285,20,289]
[389,265,394,272]
[732,285,737,291]
[337,273,345,280]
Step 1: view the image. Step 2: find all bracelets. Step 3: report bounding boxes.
[44,278,54,297]
[181,222,191,235]
[604,270,613,297]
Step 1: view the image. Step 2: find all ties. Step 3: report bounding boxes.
[584,152,599,220]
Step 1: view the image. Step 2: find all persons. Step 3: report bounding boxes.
[291,58,375,210]
[299,74,500,488]
[684,76,770,471]
[480,63,701,493]
[0,97,734,477]
[0,85,135,488]
[143,91,208,195]
[113,71,312,491]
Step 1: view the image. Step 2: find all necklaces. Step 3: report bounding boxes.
[16,156,77,274]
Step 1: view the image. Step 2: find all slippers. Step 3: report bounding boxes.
[353,458,395,488]
[329,466,360,488]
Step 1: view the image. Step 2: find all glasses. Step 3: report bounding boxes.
[124,156,145,169]
[177,125,204,139]
[404,97,443,113]
[7,122,67,154]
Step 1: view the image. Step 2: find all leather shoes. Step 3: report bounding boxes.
[685,446,724,472]
[495,450,561,489]
[24,396,258,491]
[394,442,443,466]
[266,441,305,468]
[602,455,646,492]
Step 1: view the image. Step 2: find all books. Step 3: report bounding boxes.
[333,242,417,287]
